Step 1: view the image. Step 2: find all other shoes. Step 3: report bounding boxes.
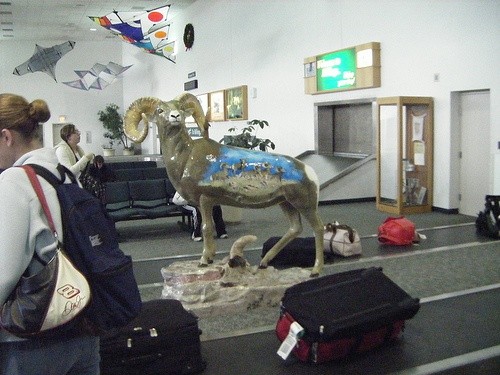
[220,234,228,238]
[191,232,202,242]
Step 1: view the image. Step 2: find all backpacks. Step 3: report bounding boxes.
[378,217,418,247]
[24,162,142,336]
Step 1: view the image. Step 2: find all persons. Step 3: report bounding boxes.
[0,92,102,375]
[55,124,95,180]
[89,155,116,183]
[172,190,229,241]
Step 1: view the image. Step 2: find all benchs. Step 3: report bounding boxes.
[87,161,195,237]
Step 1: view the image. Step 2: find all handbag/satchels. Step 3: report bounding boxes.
[261,237,318,269]
[475,210,500,238]
[0,166,91,340]
[79,154,105,201]
[313,220,362,256]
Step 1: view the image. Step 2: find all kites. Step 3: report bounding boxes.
[86,3,177,64]
[61,62,134,90]
[12,40,77,84]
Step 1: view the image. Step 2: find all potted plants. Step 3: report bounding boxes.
[97,103,134,156]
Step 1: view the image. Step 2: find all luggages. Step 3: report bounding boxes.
[276,266,420,365]
[100,298,203,375]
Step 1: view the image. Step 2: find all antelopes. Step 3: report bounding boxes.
[123,92,324,277]
